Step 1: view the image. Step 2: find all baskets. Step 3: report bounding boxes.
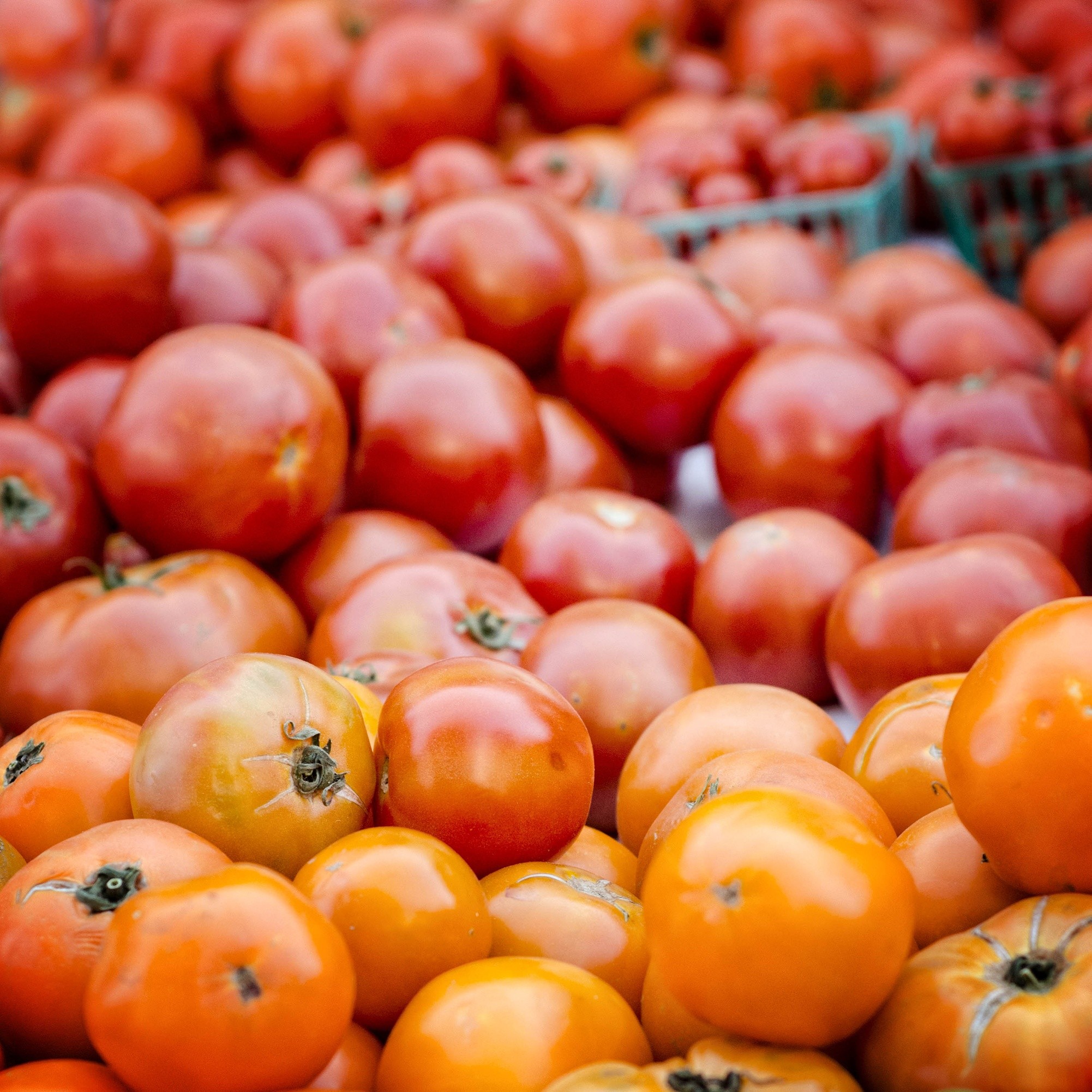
[648,111,915,256]
[909,134,1092,290]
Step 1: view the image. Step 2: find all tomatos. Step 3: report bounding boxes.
[0,0,1092,1092]
